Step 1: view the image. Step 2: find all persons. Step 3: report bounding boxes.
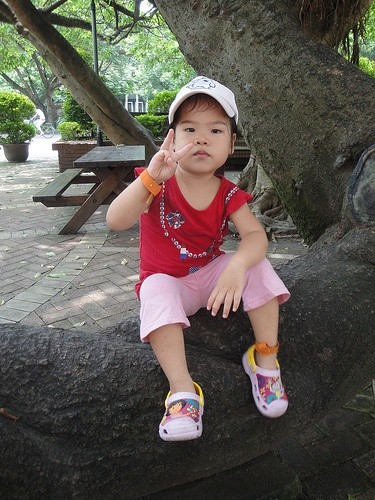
[105,76,293,441]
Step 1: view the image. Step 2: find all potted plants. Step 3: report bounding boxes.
[0,89,37,162]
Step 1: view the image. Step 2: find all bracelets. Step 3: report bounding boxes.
[138,169,164,199]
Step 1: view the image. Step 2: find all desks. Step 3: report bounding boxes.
[57,145,146,235]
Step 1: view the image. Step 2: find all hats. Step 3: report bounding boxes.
[169,75,238,126]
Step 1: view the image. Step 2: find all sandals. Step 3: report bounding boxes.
[242,344,288,417]
[159,381,204,442]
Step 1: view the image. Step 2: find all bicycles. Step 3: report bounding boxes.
[25,118,56,139]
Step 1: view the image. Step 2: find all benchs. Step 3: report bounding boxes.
[33,168,98,207]
